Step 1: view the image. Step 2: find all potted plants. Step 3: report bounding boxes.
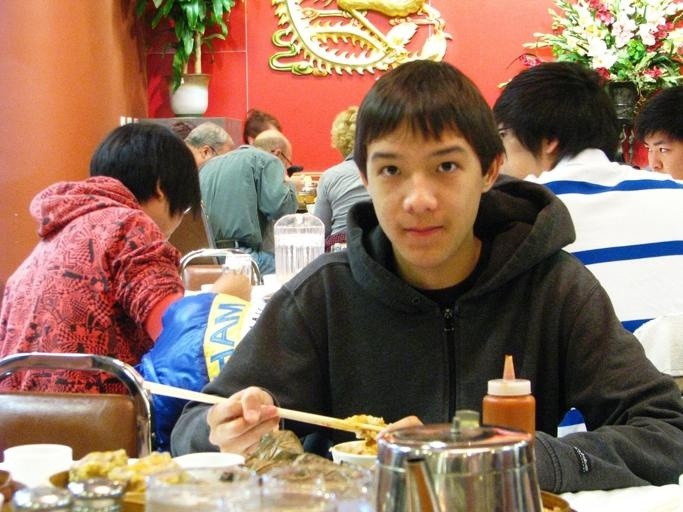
[122,0,236,118]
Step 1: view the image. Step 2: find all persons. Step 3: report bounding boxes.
[170,59,683,495]
[172,98,372,285]
[491,58,683,396]
[0,121,253,398]
[631,84,683,180]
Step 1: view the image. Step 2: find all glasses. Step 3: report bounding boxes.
[181,203,192,216]
[270,149,293,168]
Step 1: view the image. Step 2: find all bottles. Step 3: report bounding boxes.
[483,353,535,445]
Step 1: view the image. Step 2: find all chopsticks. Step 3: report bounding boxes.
[144,380,384,442]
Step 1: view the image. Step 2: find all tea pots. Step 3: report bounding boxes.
[375,424,543,512]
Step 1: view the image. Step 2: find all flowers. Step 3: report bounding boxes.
[495,0,683,111]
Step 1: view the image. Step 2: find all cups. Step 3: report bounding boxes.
[146,467,259,512]
[261,463,376,512]
[227,254,254,294]
[3,443,73,493]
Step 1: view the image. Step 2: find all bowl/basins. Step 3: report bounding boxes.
[174,451,245,479]
[329,440,378,468]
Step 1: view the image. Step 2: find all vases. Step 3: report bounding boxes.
[604,79,639,127]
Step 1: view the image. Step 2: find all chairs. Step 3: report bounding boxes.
[169,202,247,265]
[177,248,264,296]
[0,352,151,463]
